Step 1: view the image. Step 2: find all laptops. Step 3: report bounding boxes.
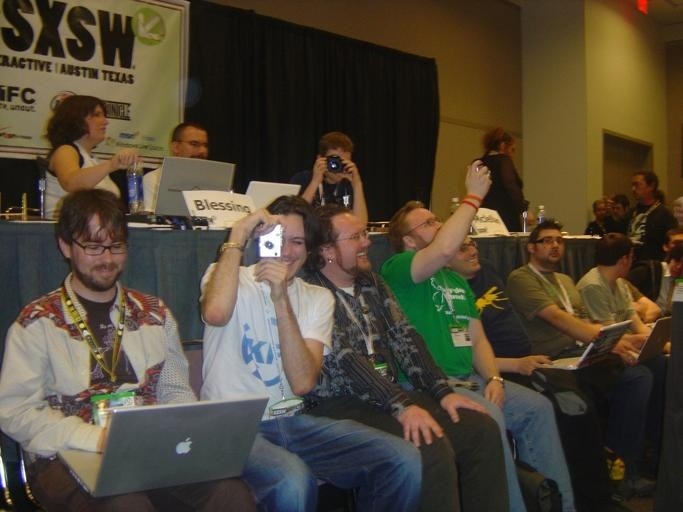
[626,317,672,365]
[535,320,632,372]
[244,179,302,210]
[154,153,236,218]
[54,395,270,497]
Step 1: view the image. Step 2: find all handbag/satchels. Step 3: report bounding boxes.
[517,463,563,512]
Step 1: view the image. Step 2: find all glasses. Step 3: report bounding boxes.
[535,236,563,244]
[323,229,369,243]
[407,216,441,236]
[460,239,481,252]
[70,238,129,256]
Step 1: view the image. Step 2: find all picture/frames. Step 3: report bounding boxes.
[0,0,190,164]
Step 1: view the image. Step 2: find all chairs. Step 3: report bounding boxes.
[185,350,204,401]
[316,479,324,485]
[0,432,42,512]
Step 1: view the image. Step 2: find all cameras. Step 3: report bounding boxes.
[327,156,345,173]
[258,223,281,258]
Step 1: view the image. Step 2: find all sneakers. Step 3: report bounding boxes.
[616,474,658,497]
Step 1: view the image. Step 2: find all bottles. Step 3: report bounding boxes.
[126,163,148,214]
[535,203,545,224]
[448,195,460,215]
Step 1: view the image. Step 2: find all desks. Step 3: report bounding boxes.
[296,233,601,287]
[0,222,261,350]
